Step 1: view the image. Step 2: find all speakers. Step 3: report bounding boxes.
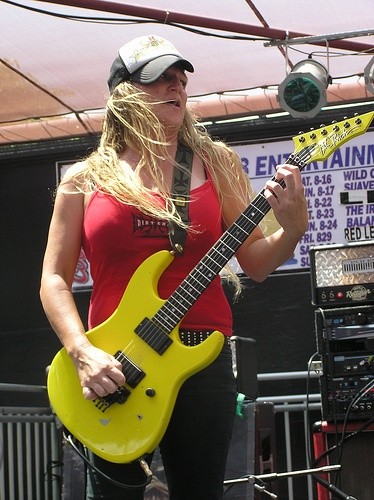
[66,401,280,500]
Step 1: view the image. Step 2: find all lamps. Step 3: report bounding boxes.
[277,59,334,120]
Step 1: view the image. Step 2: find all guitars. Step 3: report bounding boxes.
[45,110,374,465]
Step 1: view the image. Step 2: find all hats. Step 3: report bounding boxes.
[107,36,194,95]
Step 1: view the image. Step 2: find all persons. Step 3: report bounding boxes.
[37,33,308,500]
